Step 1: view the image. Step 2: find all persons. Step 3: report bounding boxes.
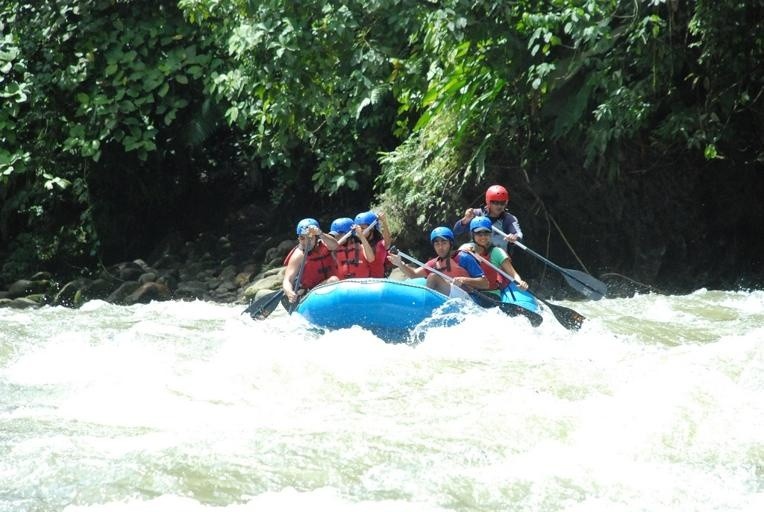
[282,218,341,304]
[387,226,489,308]
[457,217,528,301]
[356,212,390,279]
[453,185,523,257]
[328,218,375,280]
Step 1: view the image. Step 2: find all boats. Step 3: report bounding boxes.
[295,278,545,345]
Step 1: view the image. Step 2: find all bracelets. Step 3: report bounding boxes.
[400,262,408,271]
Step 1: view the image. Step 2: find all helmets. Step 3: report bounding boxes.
[296,218,319,235]
[470,217,492,232]
[331,218,354,233]
[486,185,508,208]
[431,227,454,242]
[355,213,378,225]
